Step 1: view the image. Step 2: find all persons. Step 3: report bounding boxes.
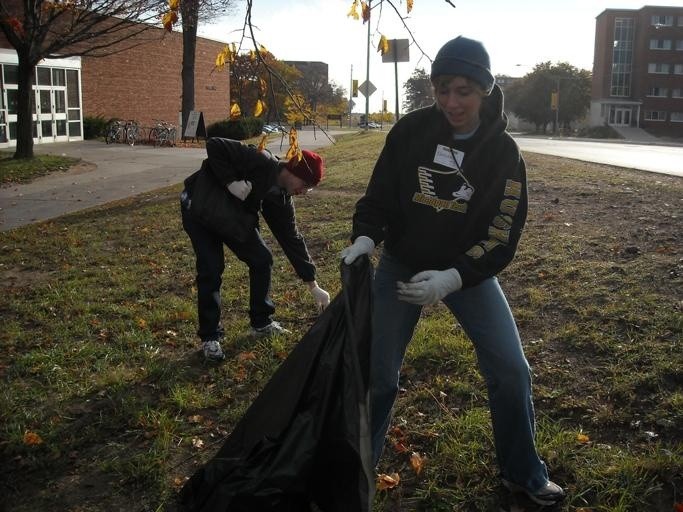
[341,36,566,505]
[180,137,330,362]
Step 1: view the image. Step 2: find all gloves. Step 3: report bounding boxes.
[226,179,252,201]
[337,236,375,265]
[396,267,462,305]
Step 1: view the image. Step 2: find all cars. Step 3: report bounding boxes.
[261,122,285,132]
[358,120,384,129]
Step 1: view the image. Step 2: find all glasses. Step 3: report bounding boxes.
[304,181,312,192]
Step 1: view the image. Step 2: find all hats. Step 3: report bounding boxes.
[286,150,323,188]
[430,35,495,96]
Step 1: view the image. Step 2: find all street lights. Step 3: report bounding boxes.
[515,63,561,137]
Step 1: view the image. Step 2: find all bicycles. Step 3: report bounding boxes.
[106,118,178,147]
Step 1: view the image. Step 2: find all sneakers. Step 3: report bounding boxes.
[203,341,225,361]
[311,285,330,307]
[251,321,292,337]
[501,479,563,505]
